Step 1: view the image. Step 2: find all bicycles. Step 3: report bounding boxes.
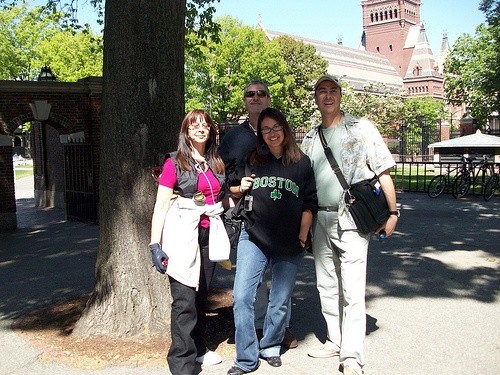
[427,156,499,202]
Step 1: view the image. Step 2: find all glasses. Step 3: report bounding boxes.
[187,122,211,130]
[244,90,270,98]
[260,125,284,134]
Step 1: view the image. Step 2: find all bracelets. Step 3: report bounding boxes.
[239,184,243,193]
[299,239,306,244]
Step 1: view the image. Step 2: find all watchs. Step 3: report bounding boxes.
[389,210,400,218]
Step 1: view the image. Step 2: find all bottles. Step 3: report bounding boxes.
[379,230,386,239]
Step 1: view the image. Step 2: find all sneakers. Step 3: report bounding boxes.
[228,328,260,344]
[283,327,298,348]
[307,344,340,357]
[343,363,363,375]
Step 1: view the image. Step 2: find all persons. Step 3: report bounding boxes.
[227,107,318,375]
[217,80,299,348]
[149,108,236,375]
[300,74,400,375]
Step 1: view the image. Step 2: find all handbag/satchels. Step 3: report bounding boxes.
[344,178,390,234]
[222,205,241,248]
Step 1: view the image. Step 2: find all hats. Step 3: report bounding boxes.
[316,75,341,90]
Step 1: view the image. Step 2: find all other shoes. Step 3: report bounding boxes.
[227,367,245,375]
[267,356,281,367]
[196,349,221,365]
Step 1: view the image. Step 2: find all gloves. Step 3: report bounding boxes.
[147,242,169,274]
